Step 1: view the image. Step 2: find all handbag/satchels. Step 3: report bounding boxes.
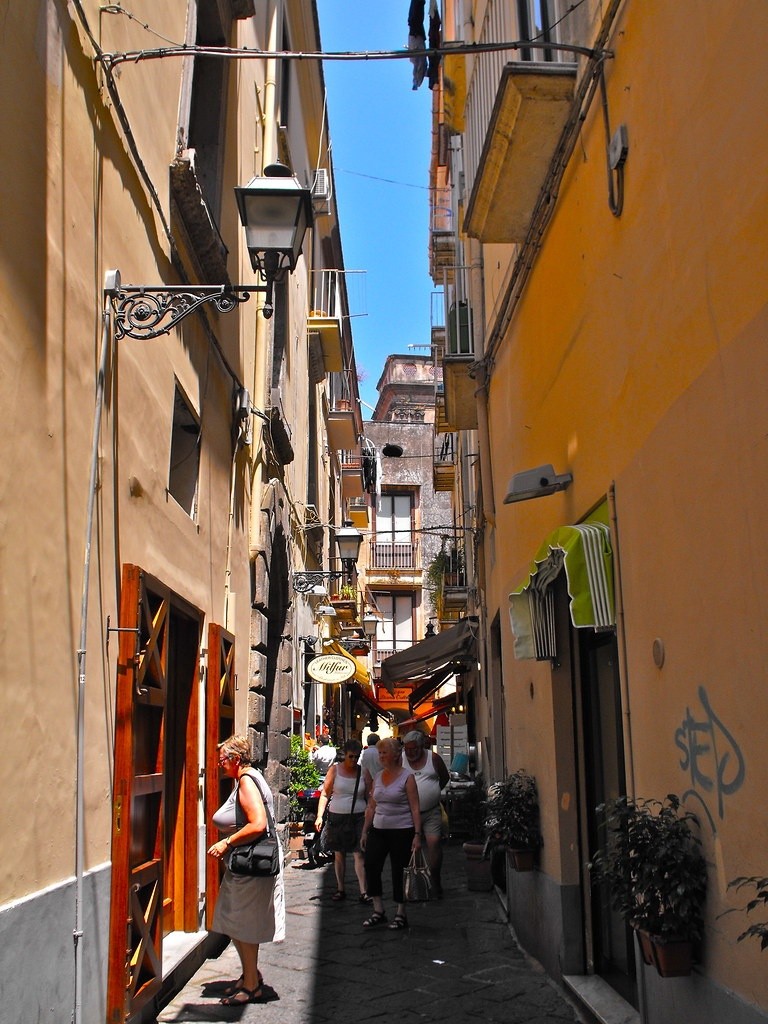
[440,802,449,827]
[401,848,438,902]
[337,821,356,851]
[229,773,280,878]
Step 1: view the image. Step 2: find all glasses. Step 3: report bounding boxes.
[403,747,417,754]
[218,757,229,766]
[347,755,359,759]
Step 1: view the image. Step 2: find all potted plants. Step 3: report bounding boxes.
[586,797,715,977]
[428,555,465,586]
[450,784,496,892]
[476,768,544,869]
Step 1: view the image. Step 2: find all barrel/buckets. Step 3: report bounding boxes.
[450,752,469,774]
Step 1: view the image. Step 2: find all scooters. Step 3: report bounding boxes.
[296,787,333,868]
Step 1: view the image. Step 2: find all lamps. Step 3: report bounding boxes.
[110,159,316,341]
[291,519,363,590]
[335,607,384,650]
[502,464,573,506]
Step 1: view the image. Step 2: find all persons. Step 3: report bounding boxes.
[357,733,384,779]
[359,738,421,929]
[401,731,449,900]
[309,735,336,775]
[315,739,373,904]
[206,734,285,1006]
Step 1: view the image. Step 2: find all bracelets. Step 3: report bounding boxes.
[415,832,421,835]
[226,836,233,848]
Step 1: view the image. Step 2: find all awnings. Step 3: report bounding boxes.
[325,637,377,698]
[379,615,478,725]
[358,693,392,725]
[507,520,615,661]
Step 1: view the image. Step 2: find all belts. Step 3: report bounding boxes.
[420,803,439,813]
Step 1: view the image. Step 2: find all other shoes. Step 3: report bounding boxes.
[431,877,443,894]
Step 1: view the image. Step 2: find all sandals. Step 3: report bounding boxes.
[363,910,387,925]
[221,984,263,1005]
[225,973,264,996]
[389,913,408,929]
[359,891,374,904]
[333,891,345,901]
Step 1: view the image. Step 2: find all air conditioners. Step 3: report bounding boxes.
[313,168,330,214]
